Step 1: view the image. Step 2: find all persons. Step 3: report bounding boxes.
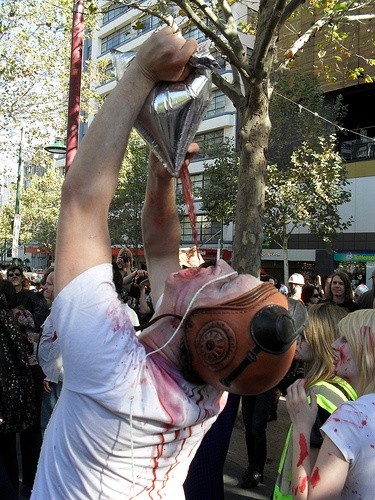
[30,26,311,500]
[0,258,375,500]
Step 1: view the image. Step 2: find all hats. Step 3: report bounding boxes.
[180,282,309,395]
[288,273,305,284]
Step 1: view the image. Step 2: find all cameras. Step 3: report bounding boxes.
[119,256,128,263]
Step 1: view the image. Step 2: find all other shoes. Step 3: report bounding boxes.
[242,471,264,488]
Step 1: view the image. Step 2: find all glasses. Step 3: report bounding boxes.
[9,273,21,277]
[314,292,322,298]
